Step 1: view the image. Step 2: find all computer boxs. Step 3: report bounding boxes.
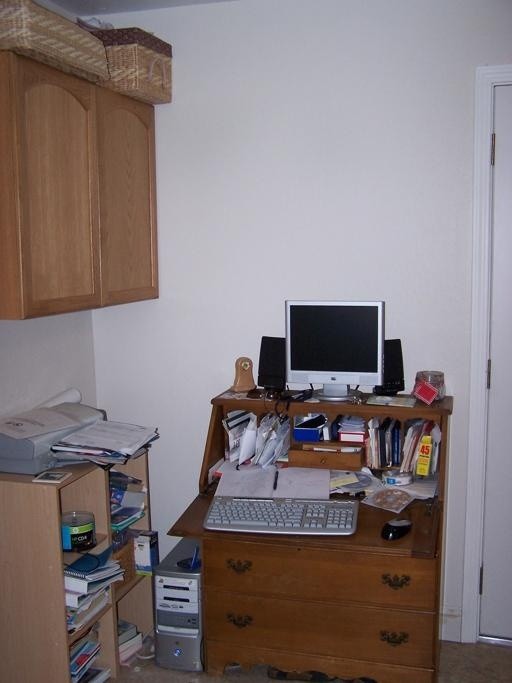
[153,537,205,673]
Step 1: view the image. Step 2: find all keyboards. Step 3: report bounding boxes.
[203,494,360,536]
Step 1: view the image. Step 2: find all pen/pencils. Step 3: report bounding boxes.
[274,471,278,489]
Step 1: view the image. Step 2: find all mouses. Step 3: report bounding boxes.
[381,516,413,540]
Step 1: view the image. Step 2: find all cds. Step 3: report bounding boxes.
[342,475,372,488]
[374,489,411,509]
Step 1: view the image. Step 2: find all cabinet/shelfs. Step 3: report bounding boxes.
[3,52,159,319]
[168,387,453,682]
[0,449,153,680]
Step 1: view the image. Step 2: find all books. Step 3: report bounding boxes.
[110,471,147,532]
[364,416,427,473]
[50,441,107,455]
[70,619,143,683]
[366,395,418,408]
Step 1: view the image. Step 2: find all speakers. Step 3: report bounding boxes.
[258,336,286,399]
[375,339,405,395]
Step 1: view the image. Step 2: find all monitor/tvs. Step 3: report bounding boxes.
[284,299,385,402]
